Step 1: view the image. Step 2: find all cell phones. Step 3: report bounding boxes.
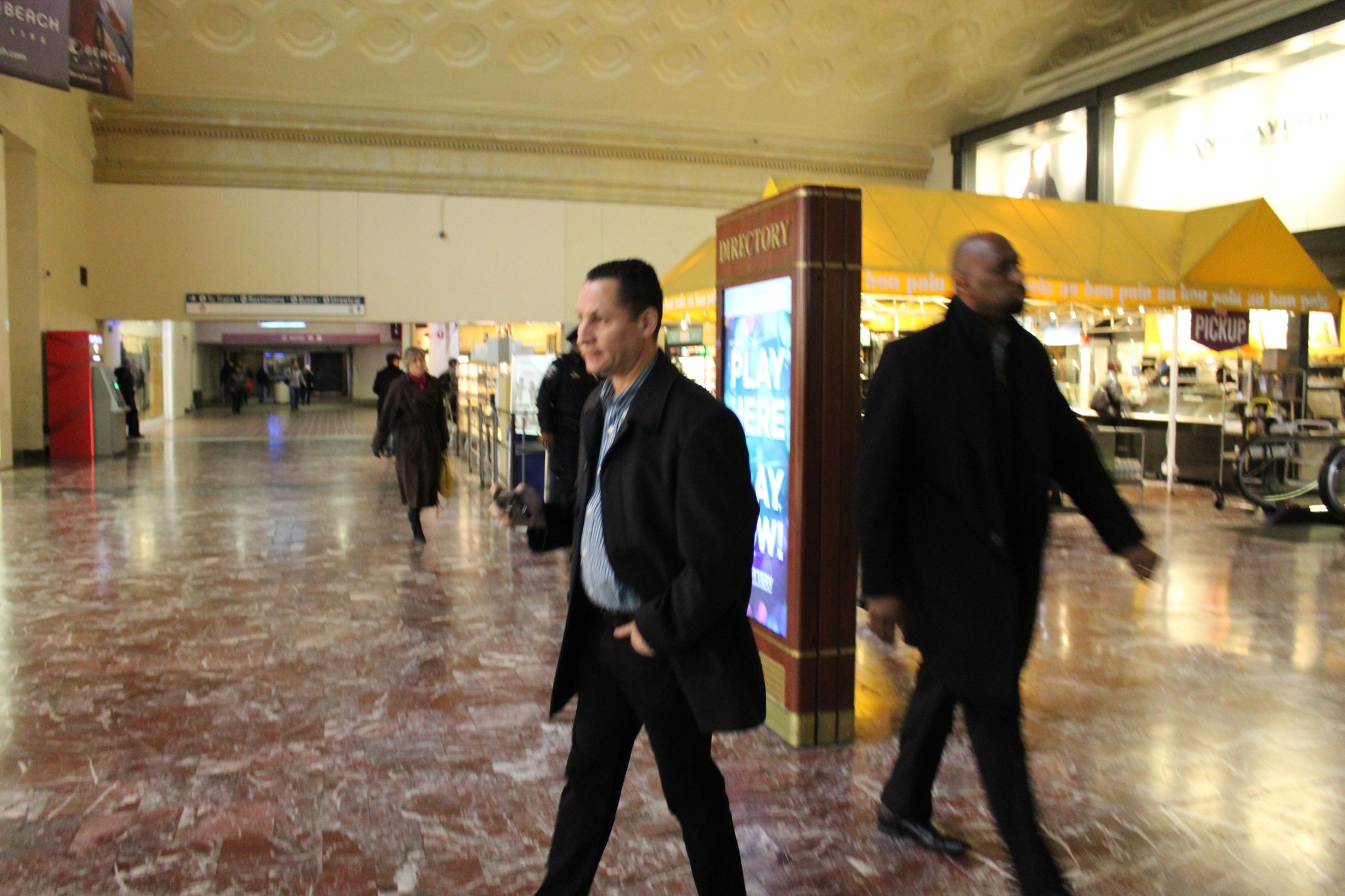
[496,489,533,520]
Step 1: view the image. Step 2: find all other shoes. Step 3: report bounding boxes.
[411,521,427,542]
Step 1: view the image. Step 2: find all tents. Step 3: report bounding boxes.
[658,177,1345,494]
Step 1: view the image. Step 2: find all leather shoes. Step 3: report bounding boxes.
[877,803,968,858]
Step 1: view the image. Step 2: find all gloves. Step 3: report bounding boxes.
[371,446,380,458]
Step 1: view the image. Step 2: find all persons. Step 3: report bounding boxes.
[439,359,458,424]
[492,258,765,896]
[860,231,1161,896]
[536,328,602,505]
[114,359,144,438]
[300,365,315,405]
[284,360,306,412]
[1103,359,1129,426]
[220,362,275,414]
[373,353,404,457]
[371,347,450,542]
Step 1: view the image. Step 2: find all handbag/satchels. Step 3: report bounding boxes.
[437,451,453,498]
